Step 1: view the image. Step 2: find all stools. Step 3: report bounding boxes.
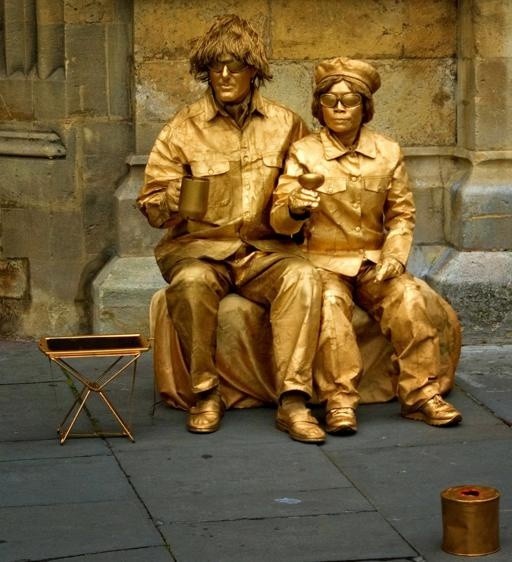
[38,331,150,444]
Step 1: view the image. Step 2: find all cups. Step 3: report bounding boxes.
[180,176,212,221]
[297,174,326,192]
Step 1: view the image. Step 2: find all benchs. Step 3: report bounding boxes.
[148,287,461,411]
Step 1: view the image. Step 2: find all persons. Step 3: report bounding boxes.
[136,11,336,444]
[266,56,465,437]
